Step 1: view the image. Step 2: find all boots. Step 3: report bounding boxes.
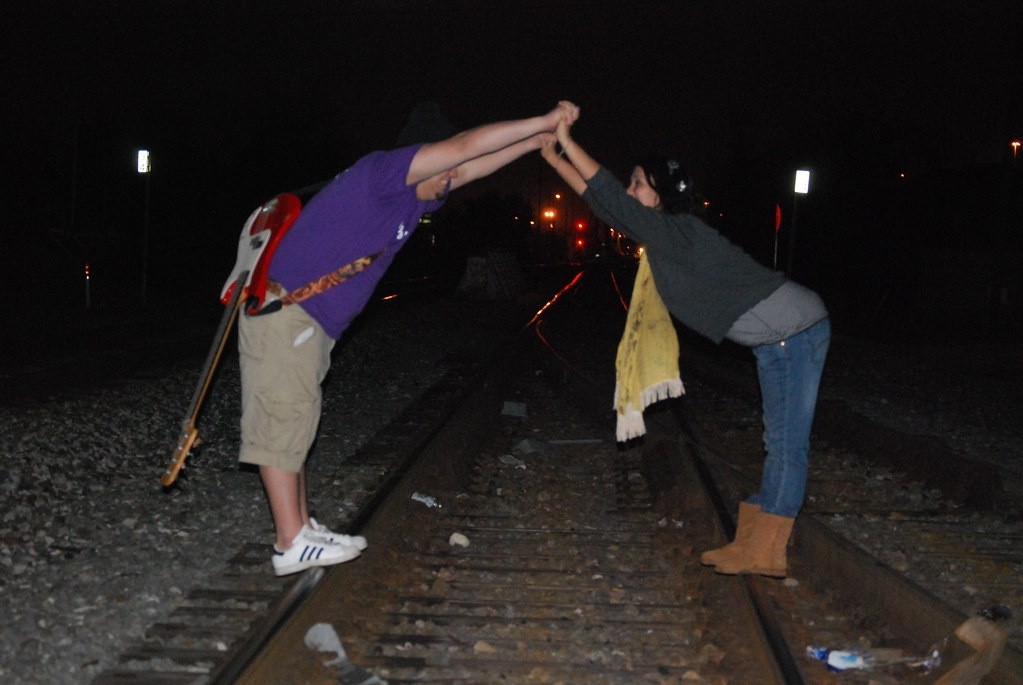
[699,502,761,568]
[712,513,797,579]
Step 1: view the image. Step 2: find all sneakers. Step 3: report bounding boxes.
[307,517,369,553]
[272,522,362,576]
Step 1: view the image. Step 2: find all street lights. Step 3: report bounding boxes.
[137,147,153,320]
[788,169,811,278]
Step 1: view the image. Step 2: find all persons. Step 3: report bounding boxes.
[239,103,579,578]
[540,100,831,579]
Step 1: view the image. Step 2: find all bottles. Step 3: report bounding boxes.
[806,644,942,675]
[404,488,456,515]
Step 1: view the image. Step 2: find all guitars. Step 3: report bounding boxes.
[161,192,301,486]
